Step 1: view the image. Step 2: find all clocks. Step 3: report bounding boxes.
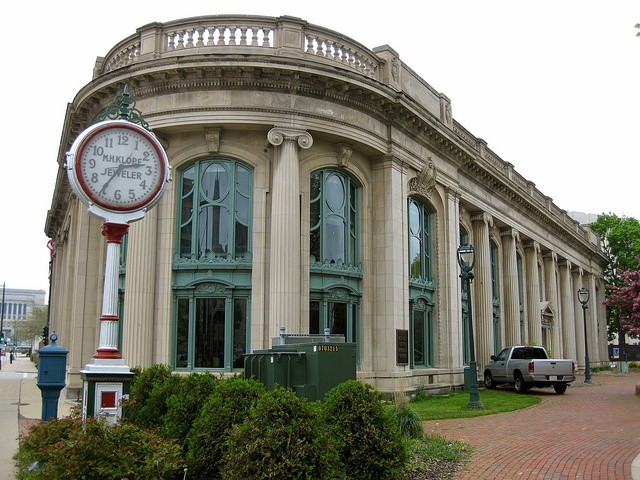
[76,123,165,211]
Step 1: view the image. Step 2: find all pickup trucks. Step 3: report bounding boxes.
[484,345,575,394]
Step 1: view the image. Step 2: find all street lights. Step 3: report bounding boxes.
[577,288,592,384]
[457,245,483,408]
